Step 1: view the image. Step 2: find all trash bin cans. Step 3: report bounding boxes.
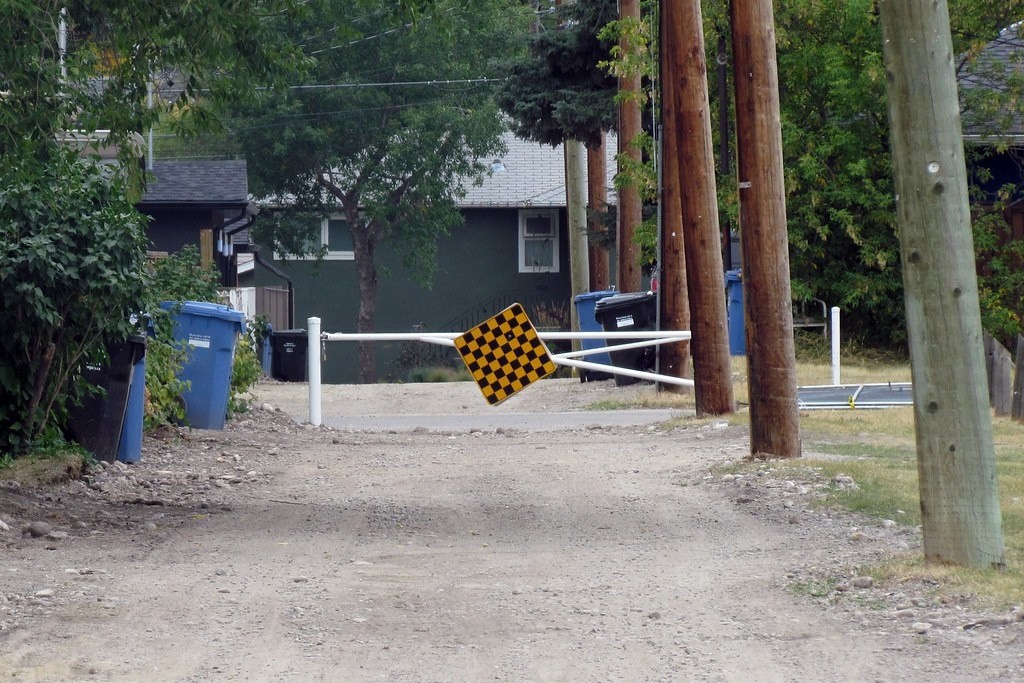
[255,323,272,373]
[159,300,246,430]
[595,293,656,386]
[270,329,309,381]
[575,290,620,383]
[724,270,746,356]
[73,332,146,462]
